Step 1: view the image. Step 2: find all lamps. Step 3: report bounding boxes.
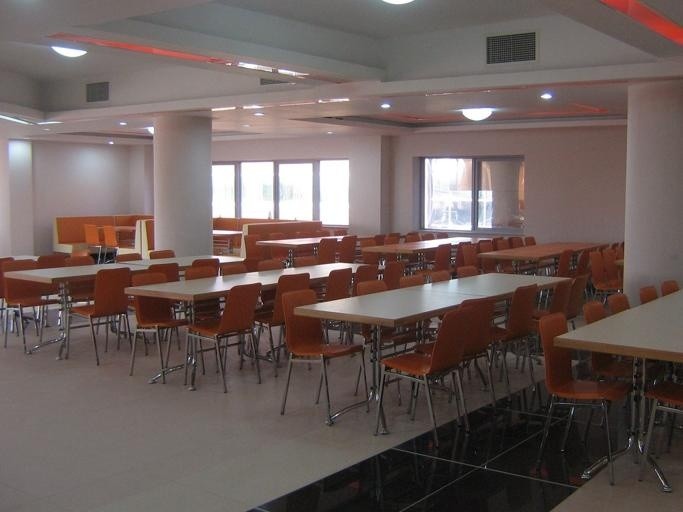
[462,107,494,121]
[50,46,88,58]
